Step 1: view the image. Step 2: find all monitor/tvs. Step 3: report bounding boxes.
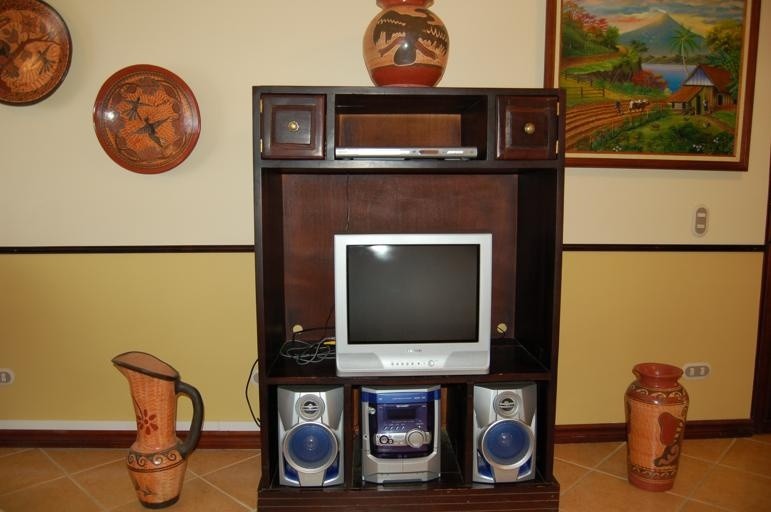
[334,233,492,378]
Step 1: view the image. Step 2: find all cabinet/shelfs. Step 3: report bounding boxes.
[253,85,566,511]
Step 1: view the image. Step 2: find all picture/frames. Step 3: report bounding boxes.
[544,0,762,171]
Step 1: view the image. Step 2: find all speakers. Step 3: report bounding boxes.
[277,385,345,488]
[472,381,538,484]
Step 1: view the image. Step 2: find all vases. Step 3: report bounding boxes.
[624,363,690,492]
[111,351,204,509]
[363,0,449,87]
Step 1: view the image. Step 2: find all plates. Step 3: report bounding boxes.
[92,64,201,173]
[2,1,72,106]
